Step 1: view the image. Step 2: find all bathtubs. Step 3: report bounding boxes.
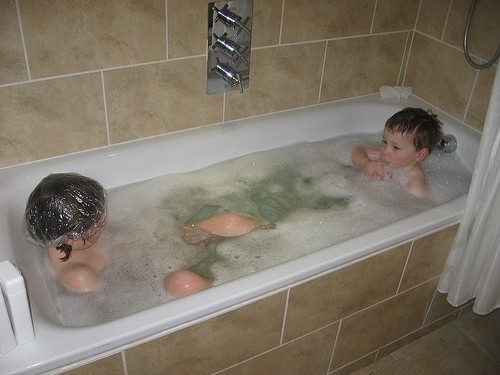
[0,84,500,375]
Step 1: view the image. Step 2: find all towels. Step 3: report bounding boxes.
[379,85,413,99]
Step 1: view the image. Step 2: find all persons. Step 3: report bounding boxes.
[23,168,260,298]
[348,104,444,199]
